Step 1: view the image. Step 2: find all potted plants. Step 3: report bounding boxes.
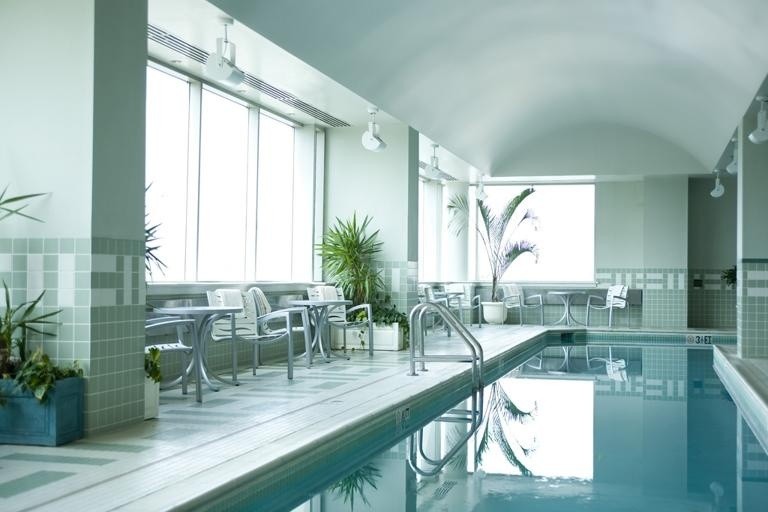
[0,181,85,447]
[314,208,410,353]
[144,347,163,420]
[446,187,540,325]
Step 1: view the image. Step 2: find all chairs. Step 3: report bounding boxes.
[585,284,631,329]
[418,284,482,338]
[205,286,312,382]
[306,286,373,364]
[585,345,630,384]
[145,316,195,396]
[511,351,544,379]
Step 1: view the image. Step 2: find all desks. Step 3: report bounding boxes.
[275,299,353,369]
[548,347,585,378]
[544,290,586,327]
[151,306,242,404]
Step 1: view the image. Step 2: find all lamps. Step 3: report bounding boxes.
[710,168,724,198]
[423,143,446,181]
[497,282,545,327]
[362,106,387,153]
[201,17,245,89]
[725,138,738,176]
[747,95,768,145]
[473,172,488,201]
